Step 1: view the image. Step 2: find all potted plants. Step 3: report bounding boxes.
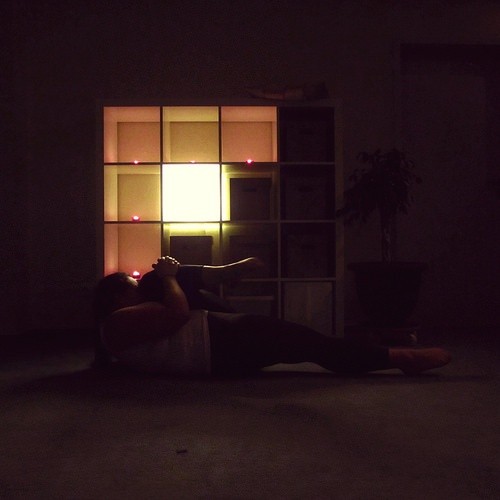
[349,146,424,325]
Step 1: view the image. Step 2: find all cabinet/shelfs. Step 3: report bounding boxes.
[91,96,346,344]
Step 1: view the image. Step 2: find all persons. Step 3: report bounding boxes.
[93,254,458,378]
